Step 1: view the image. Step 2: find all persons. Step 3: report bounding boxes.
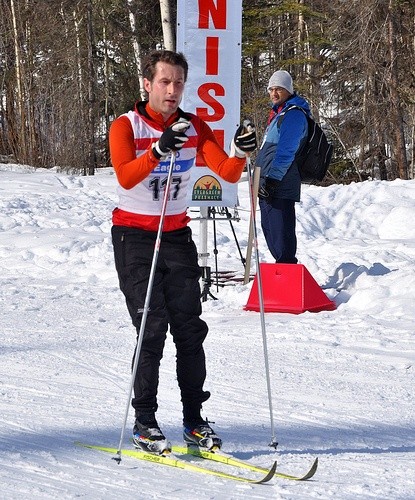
[253,69,311,264]
[112,50,258,461]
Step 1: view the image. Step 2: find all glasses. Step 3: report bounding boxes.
[268,88,283,93]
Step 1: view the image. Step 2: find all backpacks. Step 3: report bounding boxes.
[278,105,334,182]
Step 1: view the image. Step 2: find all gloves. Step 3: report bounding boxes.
[258,178,280,204]
[232,121,259,152]
[153,120,194,162]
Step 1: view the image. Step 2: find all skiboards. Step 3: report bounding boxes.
[77,432,323,486]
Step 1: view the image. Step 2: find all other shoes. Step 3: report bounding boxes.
[182,419,223,451]
[131,422,173,457]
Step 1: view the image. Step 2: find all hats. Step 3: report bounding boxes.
[266,70,300,95]
